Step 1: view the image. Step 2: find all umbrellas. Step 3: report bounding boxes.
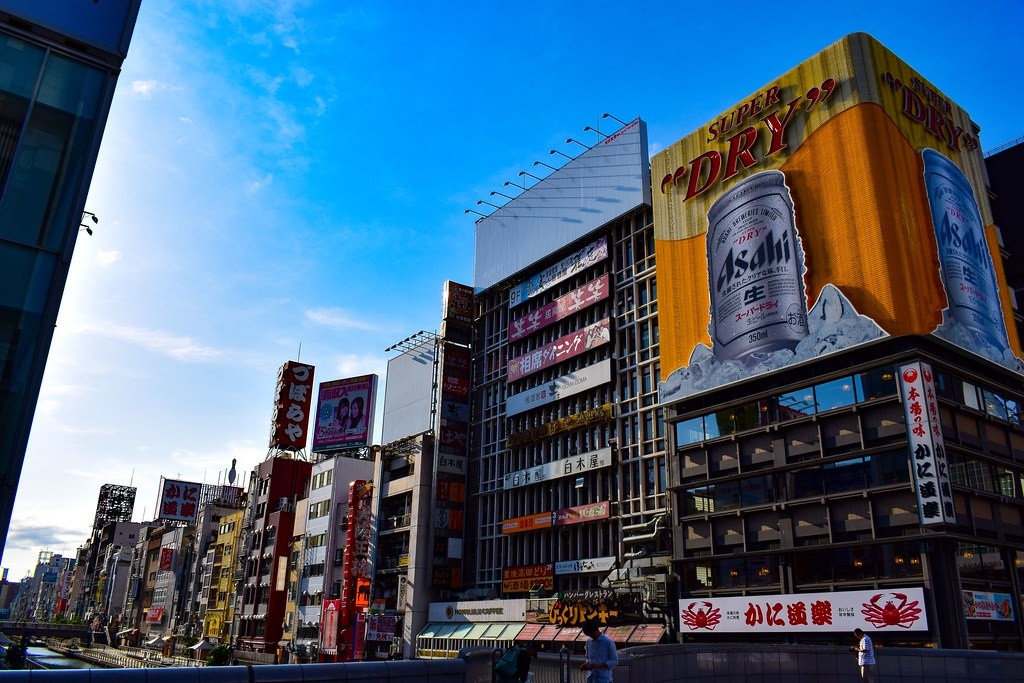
[116,628,136,646]
[186,639,213,660]
[145,635,164,667]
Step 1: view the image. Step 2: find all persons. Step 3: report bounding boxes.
[494,643,539,683]
[337,397,366,432]
[578,621,619,683]
[851,628,877,683]
[585,325,610,349]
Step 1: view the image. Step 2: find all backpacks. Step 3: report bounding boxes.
[496,646,528,680]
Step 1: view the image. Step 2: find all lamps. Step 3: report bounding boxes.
[476,200,500,210]
[464,208,487,220]
[83,210,99,224]
[532,160,558,172]
[565,138,591,151]
[778,395,821,411]
[548,149,573,164]
[390,343,408,349]
[418,329,440,339]
[384,347,402,352]
[601,112,627,129]
[398,341,415,346]
[489,190,514,200]
[404,337,422,343]
[583,126,609,141]
[411,333,429,341]
[80,223,93,237]
[518,171,541,183]
[502,181,529,191]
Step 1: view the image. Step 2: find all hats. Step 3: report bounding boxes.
[526,642,539,660]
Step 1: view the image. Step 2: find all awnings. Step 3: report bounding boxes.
[417,622,666,642]
